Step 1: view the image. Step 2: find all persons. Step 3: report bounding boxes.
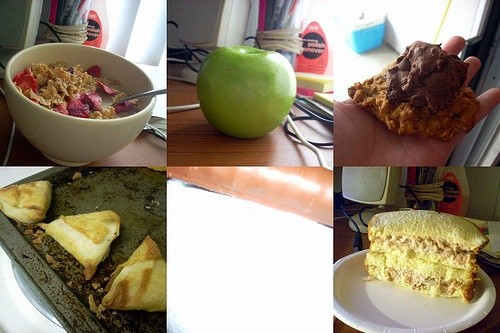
[333,34,500,166]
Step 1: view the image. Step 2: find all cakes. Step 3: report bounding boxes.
[363,209,490,303]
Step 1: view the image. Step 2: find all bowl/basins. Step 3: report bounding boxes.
[3,42,157,166]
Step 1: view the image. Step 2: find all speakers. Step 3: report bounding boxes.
[342,166,402,234]
[0,1,43,79]
[178,0,251,84]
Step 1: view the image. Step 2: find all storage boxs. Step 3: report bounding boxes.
[334,13,386,55]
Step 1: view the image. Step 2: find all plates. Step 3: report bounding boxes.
[334,249,497,333]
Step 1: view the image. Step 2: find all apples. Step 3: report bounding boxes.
[196,44,298,141]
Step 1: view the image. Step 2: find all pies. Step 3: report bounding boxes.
[1,181,53,225]
[45,209,122,281]
[101,235,166,311]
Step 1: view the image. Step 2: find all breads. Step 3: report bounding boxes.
[348,40,481,141]
